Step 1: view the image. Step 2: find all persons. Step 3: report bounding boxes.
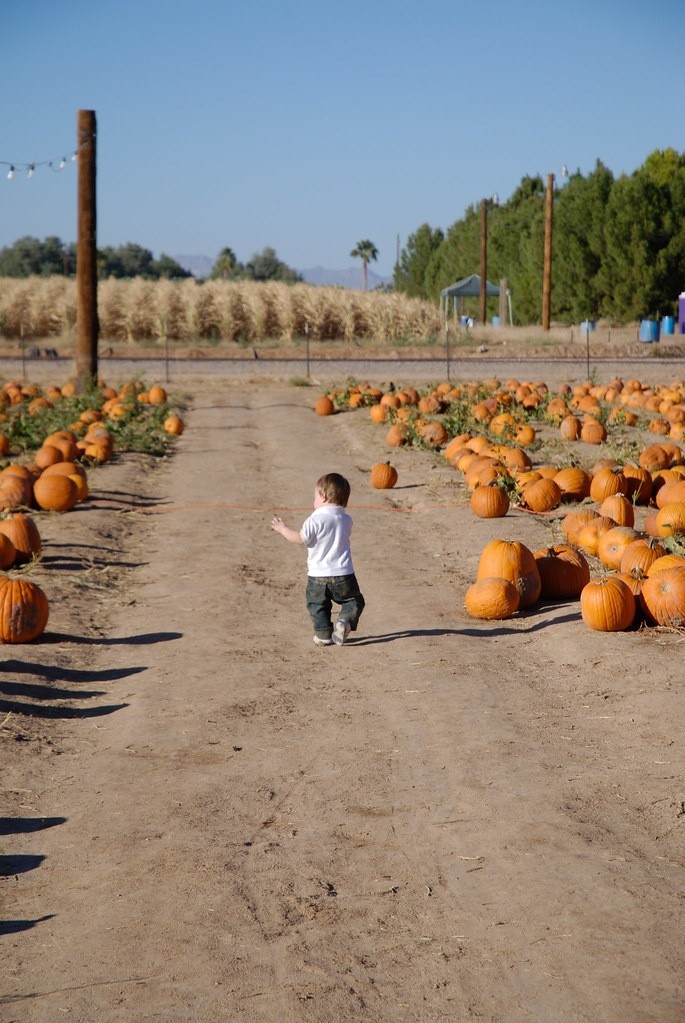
[467,317,477,328]
[492,313,500,329]
[271,473,365,645]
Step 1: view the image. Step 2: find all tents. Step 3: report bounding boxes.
[440,273,514,327]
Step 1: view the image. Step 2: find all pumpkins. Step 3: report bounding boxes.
[0,379,185,643]
[315,377,685,633]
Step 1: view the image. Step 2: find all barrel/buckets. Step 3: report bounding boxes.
[639,320,660,342]
[661,315,675,335]
[461,315,476,328]
[581,320,594,336]
[492,316,499,328]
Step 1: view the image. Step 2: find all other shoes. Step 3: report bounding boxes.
[313,632,332,645]
[333,618,352,645]
